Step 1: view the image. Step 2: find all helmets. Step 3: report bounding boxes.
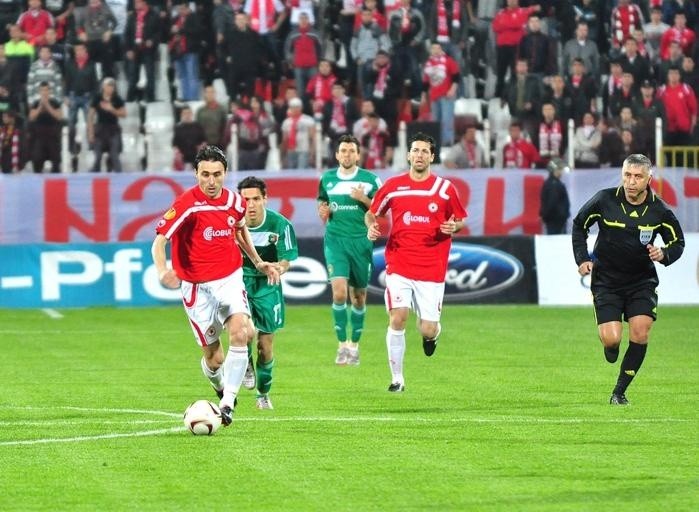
[547,157,565,172]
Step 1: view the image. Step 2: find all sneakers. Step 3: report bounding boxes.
[335,341,348,365]
[603,345,620,363]
[218,406,234,428]
[422,337,437,356]
[347,343,360,365]
[211,385,237,407]
[256,396,273,409]
[388,383,404,392]
[242,358,256,390]
[609,394,629,406]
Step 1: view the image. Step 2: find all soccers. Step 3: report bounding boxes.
[182,400,222,436]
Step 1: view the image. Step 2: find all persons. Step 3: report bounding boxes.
[539,158,571,235]
[571,154,685,405]
[352,1,389,33]
[659,39,685,84]
[502,121,541,168]
[611,130,638,167]
[609,71,644,118]
[321,80,358,167]
[534,100,568,167]
[305,60,340,120]
[276,86,297,149]
[614,36,650,84]
[363,134,469,392]
[325,23,348,84]
[492,0,541,97]
[602,59,624,120]
[610,0,646,50]
[662,0,699,31]
[617,106,647,154]
[151,145,280,428]
[392,41,417,97]
[236,175,300,413]
[559,19,601,77]
[680,56,699,99]
[643,4,670,64]
[361,112,394,169]
[567,0,601,42]
[539,13,563,70]
[389,0,426,101]
[464,0,507,80]
[574,111,602,169]
[621,25,647,57]
[597,115,622,167]
[0,0,285,173]
[517,10,550,72]
[421,41,461,147]
[443,124,492,169]
[564,57,598,128]
[655,67,699,146]
[499,58,539,148]
[630,79,667,166]
[349,10,392,98]
[281,98,317,169]
[315,135,384,366]
[428,0,470,99]
[282,11,322,98]
[659,11,696,62]
[364,49,397,121]
[542,70,572,121]
[285,0,317,29]
[352,97,389,145]
